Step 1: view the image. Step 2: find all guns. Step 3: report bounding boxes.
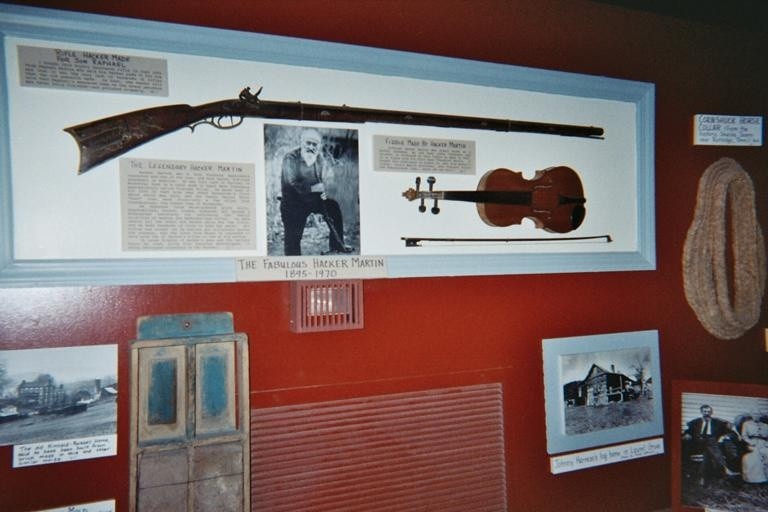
[63,86,606,176]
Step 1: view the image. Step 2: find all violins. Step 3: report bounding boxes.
[401,165,586,235]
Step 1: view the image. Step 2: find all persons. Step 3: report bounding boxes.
[278,130,355,257]
[687,405,741,486]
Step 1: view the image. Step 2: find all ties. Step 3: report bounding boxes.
[703,421,708,435]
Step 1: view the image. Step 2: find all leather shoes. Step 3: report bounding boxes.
[330,244,355,254]
[724,472,741,481]
